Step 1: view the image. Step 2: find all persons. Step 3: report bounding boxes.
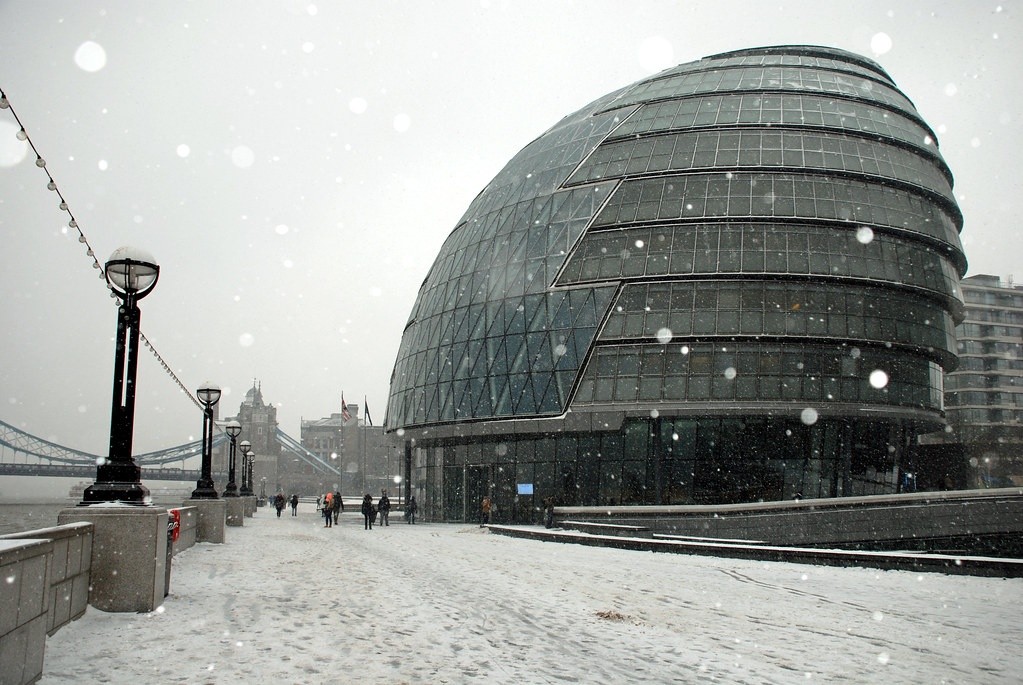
[490,499,497,522]
[405,496,418,524]
[254,495,258,507]
[546,495,555,529]
[481,494,491,524]
[290,495,299,516]
[268,493,291,517]
[317,492,391,530]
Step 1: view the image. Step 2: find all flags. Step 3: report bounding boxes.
[365,402,373,427]
[342,399,352,423]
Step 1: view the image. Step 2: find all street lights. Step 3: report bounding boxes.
[260,477,268,499]
[240,440,251,496]
[379,445,396,491]
[222,420,240,497]
[190,380,221,499]
[331,453,343,494]
[247,452,258,496]
[81,248,160,505]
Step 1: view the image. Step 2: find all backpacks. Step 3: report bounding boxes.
[327,498,334,509]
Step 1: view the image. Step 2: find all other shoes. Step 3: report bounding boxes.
[329,525,331,527]
[380,523,382,526]
[386,524,390,526]
[325,526,328,527]
